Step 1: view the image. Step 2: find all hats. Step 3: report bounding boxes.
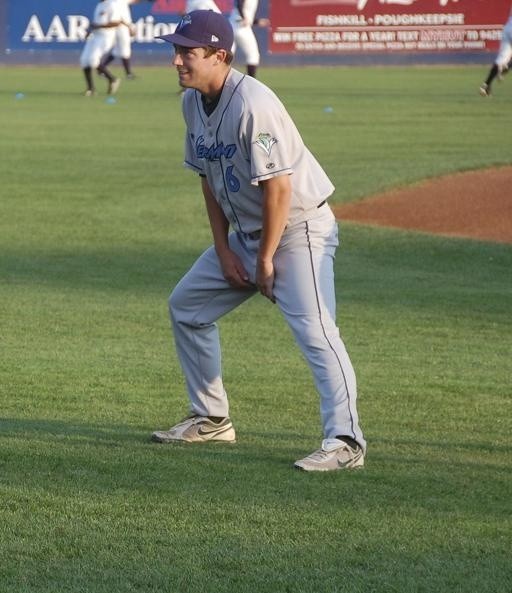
[154,11,233,50]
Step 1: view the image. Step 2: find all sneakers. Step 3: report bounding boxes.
[294,437,364,473]
[152,416,236,445]
[497,62,508,79]
[480,84,492,97]
[83,71,139,96]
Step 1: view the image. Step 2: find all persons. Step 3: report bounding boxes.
[478,5,512,96]
[80,0,124,97]
[227,0,260,80]
[152,9,367,472]
[183,1,219,15]
[97,0,142,79]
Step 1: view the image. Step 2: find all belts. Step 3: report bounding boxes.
[246,230,263,241]
